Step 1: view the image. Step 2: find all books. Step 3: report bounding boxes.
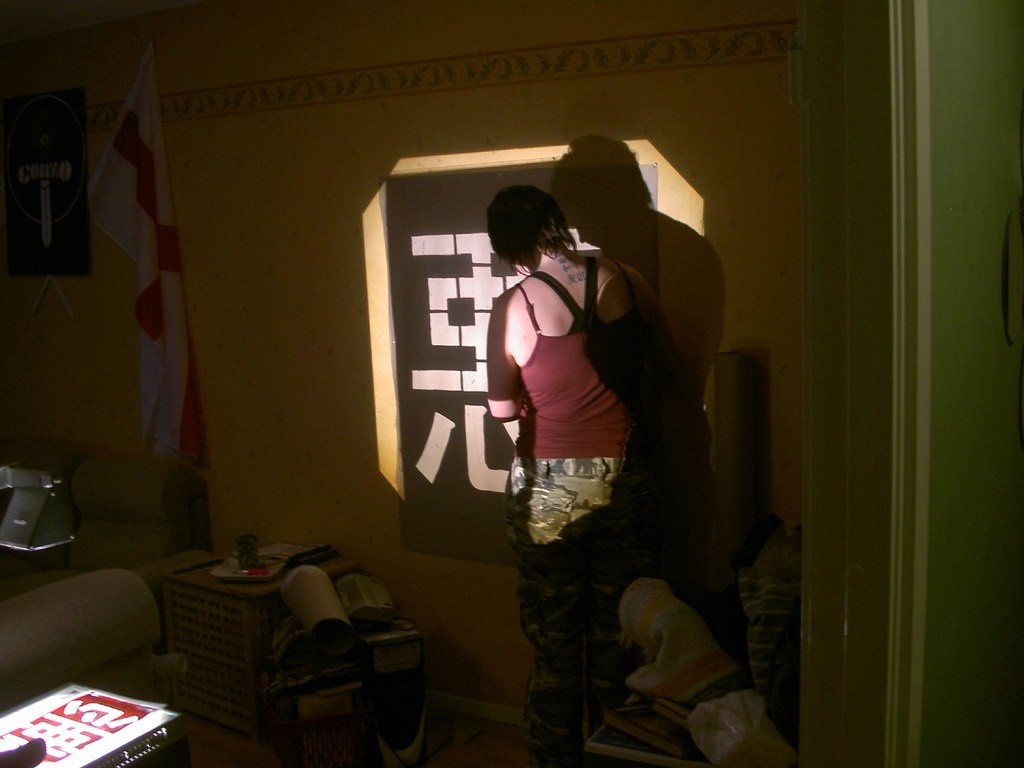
[582,723,705,768]
[651,697,692,732]
[257,616,426,721]
[601,703,699,758]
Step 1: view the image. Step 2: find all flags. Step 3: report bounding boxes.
[85,42,202,462]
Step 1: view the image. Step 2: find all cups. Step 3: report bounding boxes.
[235,535,259,573]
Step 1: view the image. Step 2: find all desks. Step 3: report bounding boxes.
[161,551,364,740]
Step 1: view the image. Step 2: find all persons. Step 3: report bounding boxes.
[486,186,672,768]
[0,735,46,768]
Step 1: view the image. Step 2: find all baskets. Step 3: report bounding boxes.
[256,681,375,768]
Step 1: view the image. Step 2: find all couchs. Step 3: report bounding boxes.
[0,445,207,707]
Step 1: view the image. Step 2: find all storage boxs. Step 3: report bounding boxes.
[247,651,376,768]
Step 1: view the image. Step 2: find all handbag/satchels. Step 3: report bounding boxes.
[337,570,417,631]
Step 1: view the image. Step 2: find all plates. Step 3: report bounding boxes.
[209,558,286,581]
